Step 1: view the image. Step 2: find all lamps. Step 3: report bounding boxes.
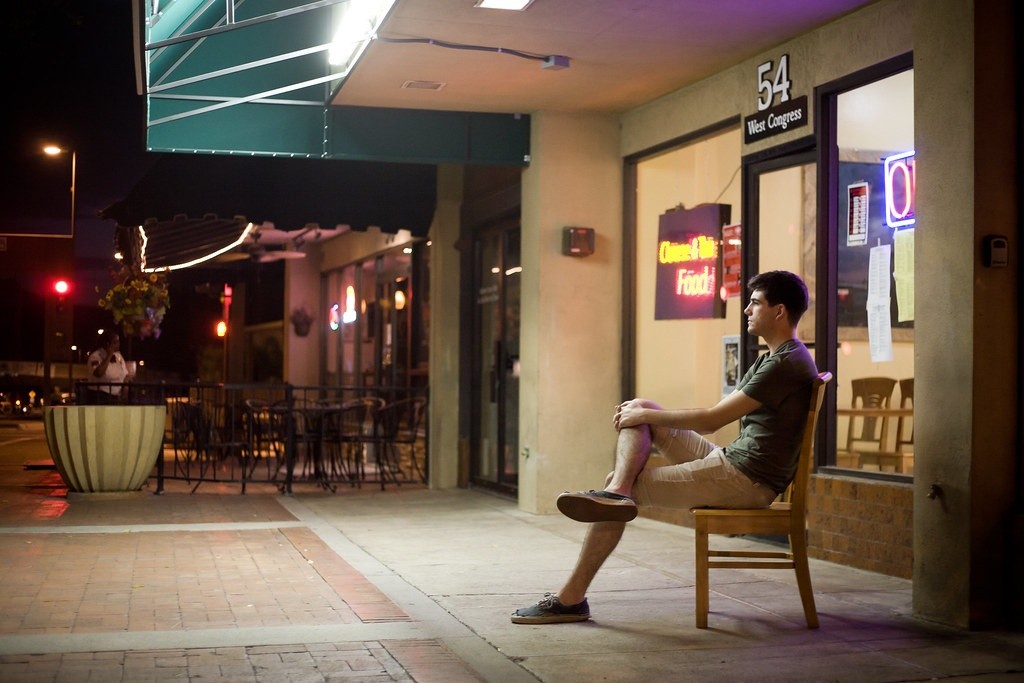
[323,12,373,67]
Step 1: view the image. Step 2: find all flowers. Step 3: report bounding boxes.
[97,274,171,341]
[289,307,315,327]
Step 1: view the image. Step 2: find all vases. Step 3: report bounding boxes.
[41,404,166,491]
[294,323,311,337]
[118,314,152,339]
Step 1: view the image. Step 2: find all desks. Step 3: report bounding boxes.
[836,407,913,418]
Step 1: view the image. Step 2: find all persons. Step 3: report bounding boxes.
[87,333,129,406]
[511,270,818,624]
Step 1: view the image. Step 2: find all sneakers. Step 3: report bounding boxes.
[510,591,592,624]
[556,489,638,523]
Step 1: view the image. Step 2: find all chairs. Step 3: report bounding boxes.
[170,396,427,495]
[858,378,914,473]
[836,376,898,473]
[691,370,836,632]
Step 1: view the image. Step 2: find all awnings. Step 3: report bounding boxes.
[148,0,531,235]
